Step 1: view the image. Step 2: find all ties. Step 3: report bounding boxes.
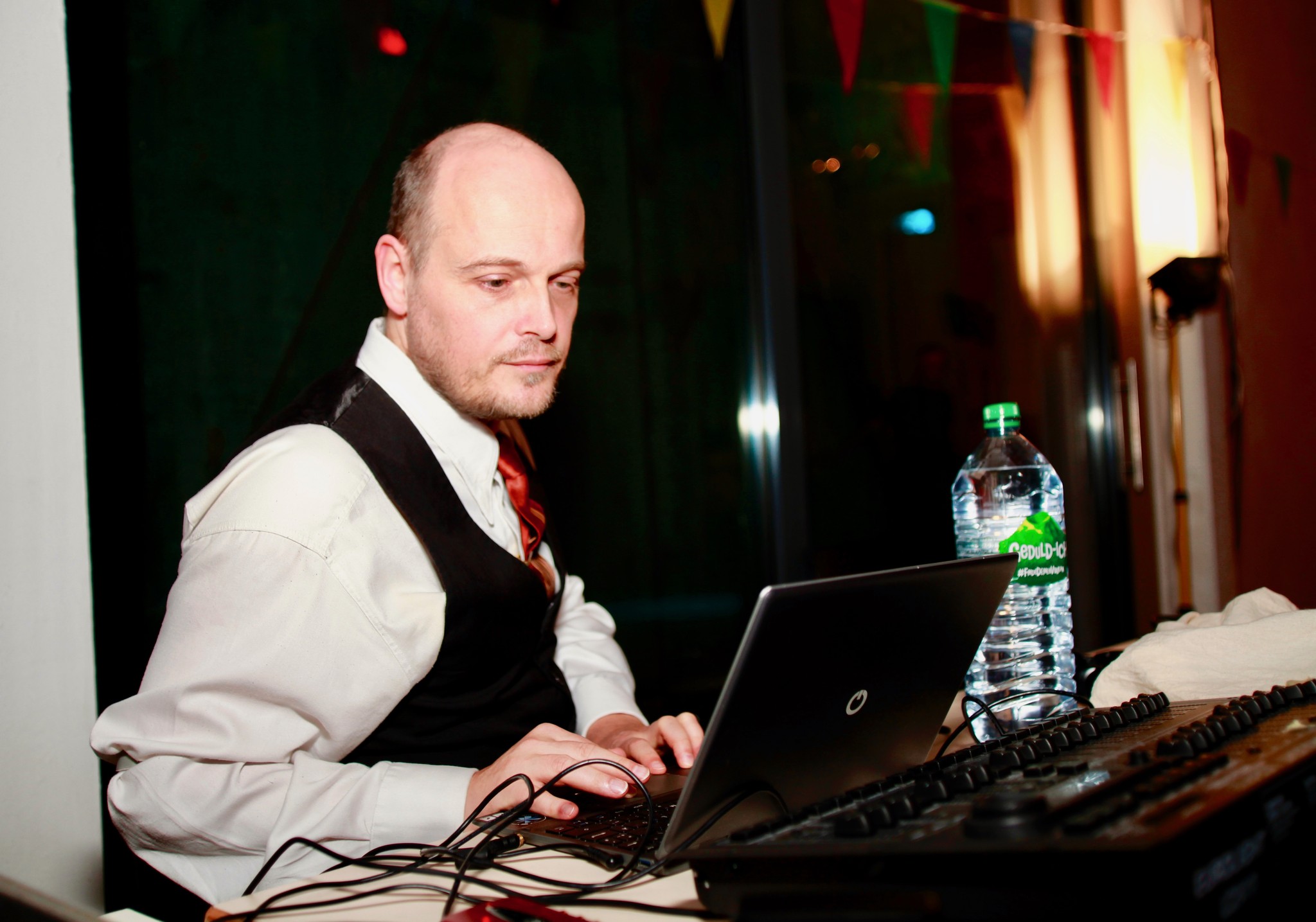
[494,430,554,597]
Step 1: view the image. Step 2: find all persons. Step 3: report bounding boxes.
[93,124,706,919]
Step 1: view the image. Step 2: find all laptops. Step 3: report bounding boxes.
[475,554,1023,873]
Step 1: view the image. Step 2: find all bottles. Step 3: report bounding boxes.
[950,402,1078,743]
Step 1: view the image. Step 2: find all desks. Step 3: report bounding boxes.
[205,635,1134,922]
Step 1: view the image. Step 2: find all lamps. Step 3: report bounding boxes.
[1148,257,1224,614]
